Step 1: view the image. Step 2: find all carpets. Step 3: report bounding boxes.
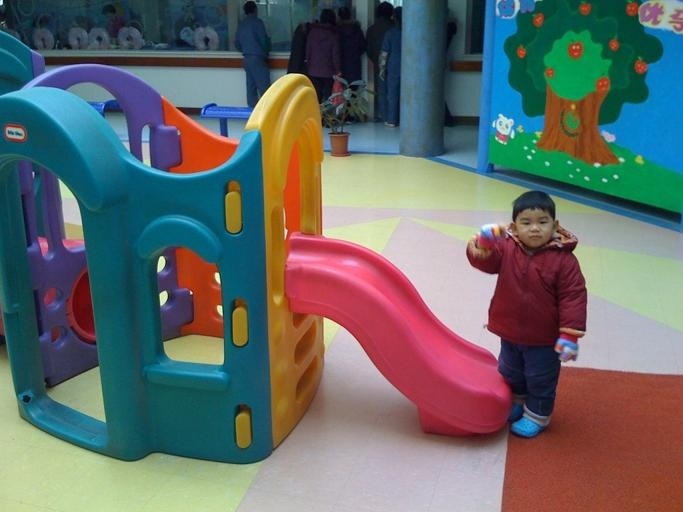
[501,366,683,512]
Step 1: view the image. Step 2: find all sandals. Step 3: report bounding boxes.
[511,394,552,438]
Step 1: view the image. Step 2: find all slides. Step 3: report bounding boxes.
[284,230,512,435]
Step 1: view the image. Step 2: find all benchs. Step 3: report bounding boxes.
[87,100,119,118]
[201,103,254,137]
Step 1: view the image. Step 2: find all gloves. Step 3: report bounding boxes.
[553,333,579,363]
[478,222,506,247]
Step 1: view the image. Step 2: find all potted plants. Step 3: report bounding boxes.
[319,75,376,156]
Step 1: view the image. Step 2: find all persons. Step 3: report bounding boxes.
[231,0,458,127]
[100,4,125,46]
[467,189,589,440]
